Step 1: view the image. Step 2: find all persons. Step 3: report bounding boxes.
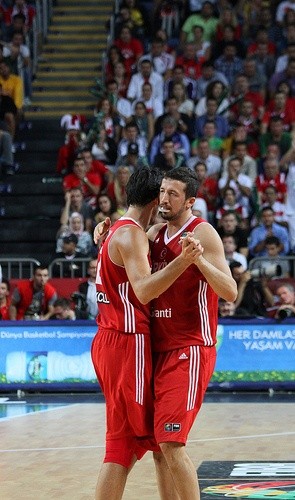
[94,166,238,500]
[91,165,204,500]
[1,0,295,321]
